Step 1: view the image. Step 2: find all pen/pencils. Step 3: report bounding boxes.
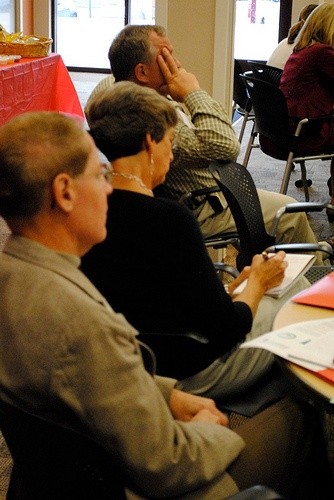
[262,251,269,261]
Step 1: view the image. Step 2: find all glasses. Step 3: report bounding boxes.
[50,163,108,210]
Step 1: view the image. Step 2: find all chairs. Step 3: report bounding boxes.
[0,59,334,500]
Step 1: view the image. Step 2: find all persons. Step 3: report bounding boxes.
[0,111,334,500]
[86,25,329,266]
[85,81,312,395]
[267,1,334,224]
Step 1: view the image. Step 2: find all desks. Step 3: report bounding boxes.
[274,271,334,500]
[0,52,85,130]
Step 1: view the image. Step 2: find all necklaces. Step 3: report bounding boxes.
[111,171,152,193]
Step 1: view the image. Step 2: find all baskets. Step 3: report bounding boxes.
[0,35,53,58]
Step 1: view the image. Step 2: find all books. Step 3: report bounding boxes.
[232,253,317,298]
[293,271,334,309]
